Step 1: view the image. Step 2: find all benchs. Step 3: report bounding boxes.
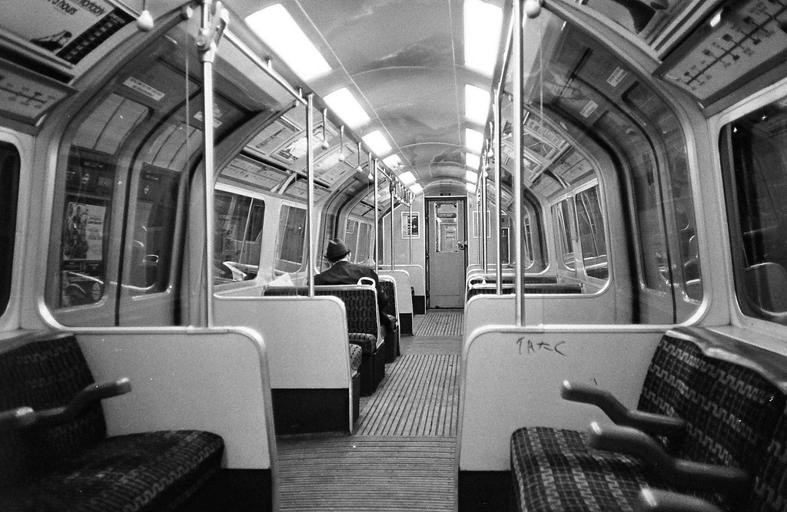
[506,329,786,511]
[463,285,585,301]
[465,276,574,286]
[0,332,226,511]
[263,279,403,422]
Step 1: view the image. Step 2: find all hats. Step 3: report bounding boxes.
[323,238,350,258]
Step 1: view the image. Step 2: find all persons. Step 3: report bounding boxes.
[64,202,90,260]
[310,239,396,365]
